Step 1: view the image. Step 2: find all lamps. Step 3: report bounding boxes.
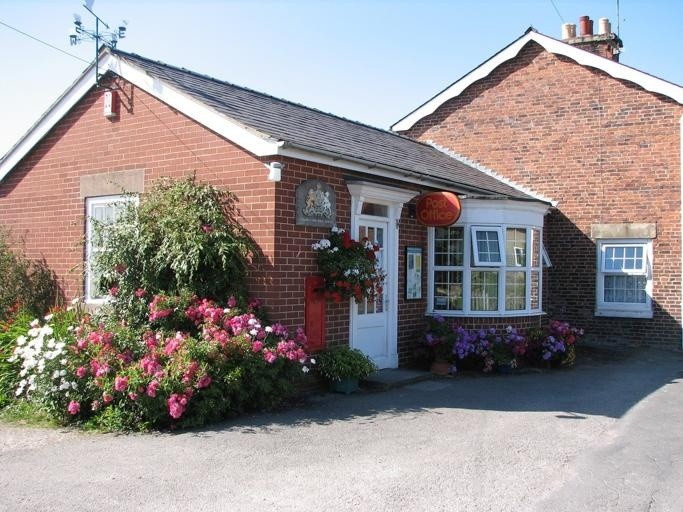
[267,160,285,184]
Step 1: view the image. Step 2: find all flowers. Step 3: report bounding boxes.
[415,314,586,378]
[311,226,387,306]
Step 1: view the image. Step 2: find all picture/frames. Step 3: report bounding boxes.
[403,244,425,304]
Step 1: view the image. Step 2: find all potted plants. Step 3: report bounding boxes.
[315,341,380,395]
